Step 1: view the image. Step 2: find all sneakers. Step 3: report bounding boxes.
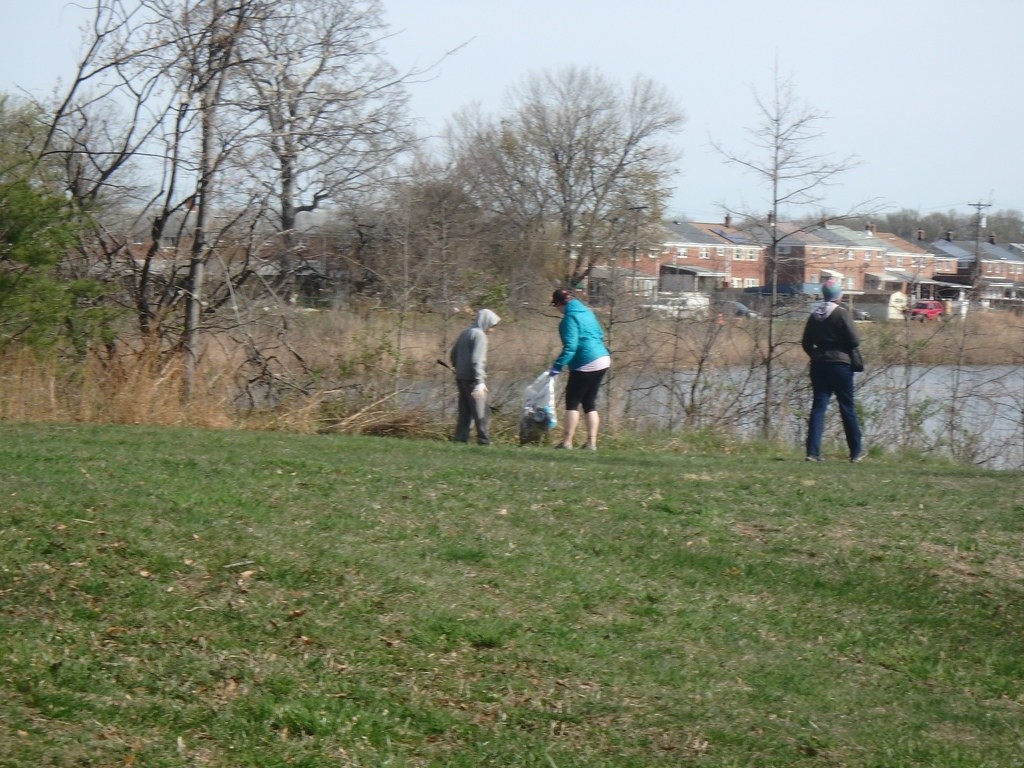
[806,453,824,462]
[851,449,869,462]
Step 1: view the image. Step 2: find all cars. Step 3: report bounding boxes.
[810,302,871,320]
[987,286,1024,314]
[903,301,944,323]
[710,301,762,319]
[638,298,703,320]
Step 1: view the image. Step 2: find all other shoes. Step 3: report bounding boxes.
[580,443,598,450]
[555,442,573,450]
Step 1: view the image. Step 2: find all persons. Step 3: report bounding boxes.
[549,289,611,450]
[802,280,868,463]
[450,308,502,445]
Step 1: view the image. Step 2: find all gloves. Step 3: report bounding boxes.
[549,368,560,376]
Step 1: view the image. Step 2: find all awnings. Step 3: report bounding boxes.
[866,273,897,281]
[821,270,844,279]
[984,279,1015,287]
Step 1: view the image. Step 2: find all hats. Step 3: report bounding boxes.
[552,289,570,305]
[823,280,844,302]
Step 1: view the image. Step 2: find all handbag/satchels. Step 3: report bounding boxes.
[851,348,863,372]
